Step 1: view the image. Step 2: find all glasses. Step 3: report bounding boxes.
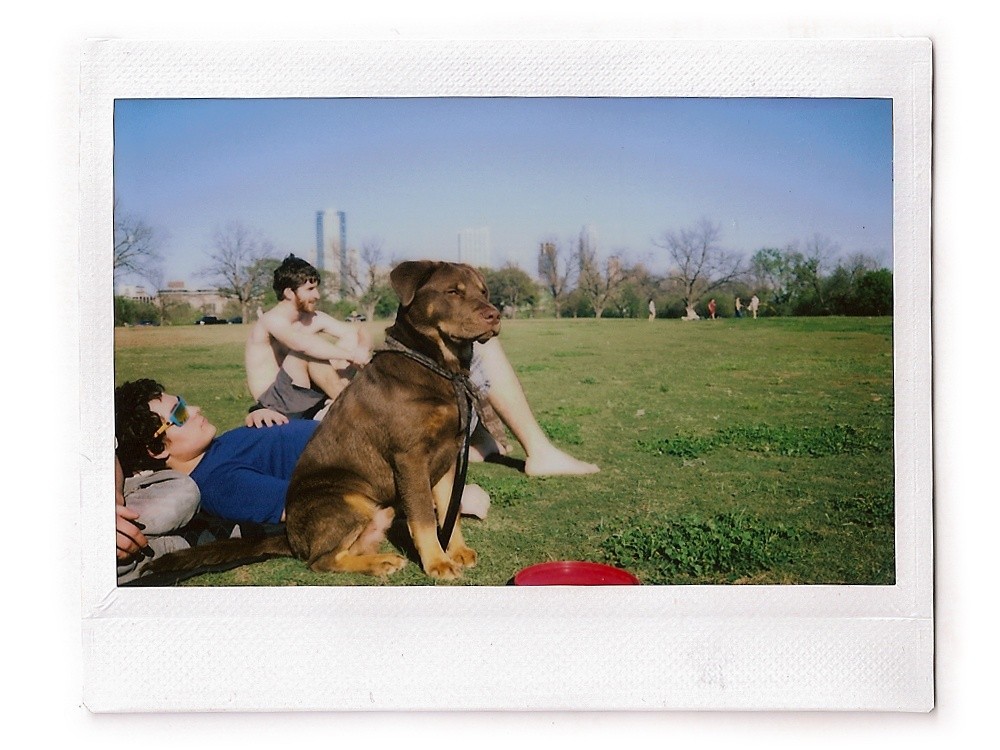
[153,395,190,438]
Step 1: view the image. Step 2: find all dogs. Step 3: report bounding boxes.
[138,259,502,581]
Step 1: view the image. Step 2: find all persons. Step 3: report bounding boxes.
[116,436,201,585]
[750,294,759,319]
[708,298,716,320]
[648,299,656,320]
[245,254,373,421]
[735,296,744,318]
[115,336,602,526]
